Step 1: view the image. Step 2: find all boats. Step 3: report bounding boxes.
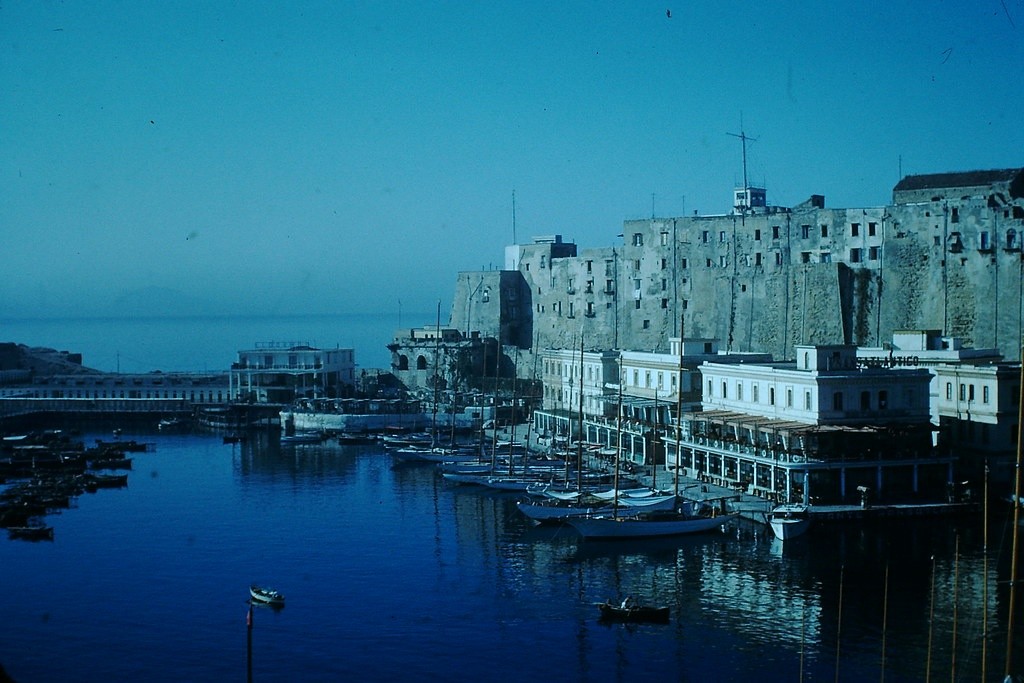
[0,426,156,542]
[768,505,811,542]
[248,583,286,605]
[157,418,182,429]
[596,600,645,622]
[222,434,247,443]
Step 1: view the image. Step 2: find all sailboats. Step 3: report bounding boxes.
[278,298,742,540]
